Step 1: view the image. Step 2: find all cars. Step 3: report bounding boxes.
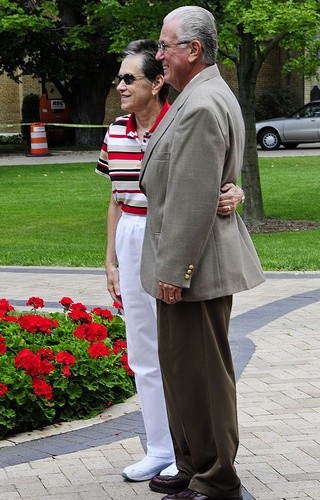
[255,99,320,151]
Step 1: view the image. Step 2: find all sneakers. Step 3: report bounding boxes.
[159,463,179,476]
[122,455,174,482]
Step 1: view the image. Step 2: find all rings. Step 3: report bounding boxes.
[227,206,230,211]
[169,296,175,300]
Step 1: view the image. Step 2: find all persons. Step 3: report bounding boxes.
[139,6,265,500]
[95,40,245,482]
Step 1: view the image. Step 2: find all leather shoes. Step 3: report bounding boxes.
[149,472,189,493]
[162,489,243,500]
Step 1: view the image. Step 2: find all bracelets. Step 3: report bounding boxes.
[236,186,246,204]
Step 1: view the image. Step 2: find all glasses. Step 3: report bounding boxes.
[112,73,149,86]
[157,38,204,54]
